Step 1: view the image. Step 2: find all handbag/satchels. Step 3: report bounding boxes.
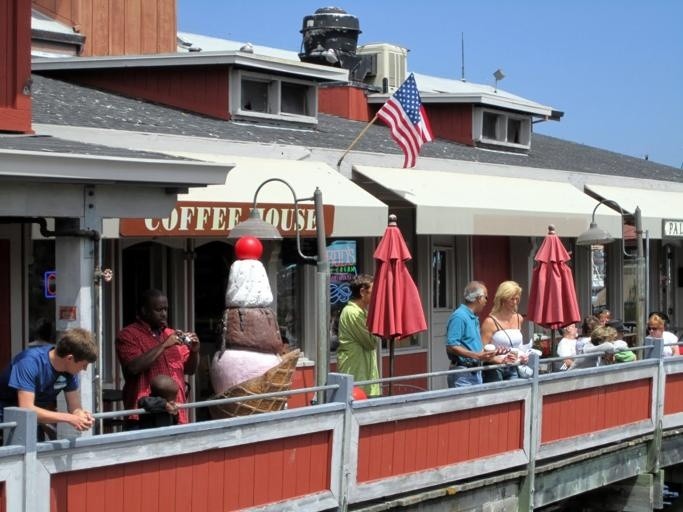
[517,364,533,378]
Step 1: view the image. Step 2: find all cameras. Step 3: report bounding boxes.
[175,328,193,346]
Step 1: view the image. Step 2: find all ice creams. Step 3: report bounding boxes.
[208,237,301,419]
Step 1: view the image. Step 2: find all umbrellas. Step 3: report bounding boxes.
[526,224,582,373]
[366,214,427,395]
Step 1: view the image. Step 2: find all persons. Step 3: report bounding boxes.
[554,308,638,372]
[136,374,178,428]
[445,280,510,387]
[480,280,529,383]
[646,313,680,358]
[0,327,99,442]
[336,274,383,398]
[113,287,200,432]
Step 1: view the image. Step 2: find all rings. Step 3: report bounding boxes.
[176,337,179,339]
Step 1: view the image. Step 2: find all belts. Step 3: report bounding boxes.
[451,360,478,366]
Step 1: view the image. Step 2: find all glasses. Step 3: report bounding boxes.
[648,327,657,330]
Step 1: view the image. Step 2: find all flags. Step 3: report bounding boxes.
[375,73,434,168]
